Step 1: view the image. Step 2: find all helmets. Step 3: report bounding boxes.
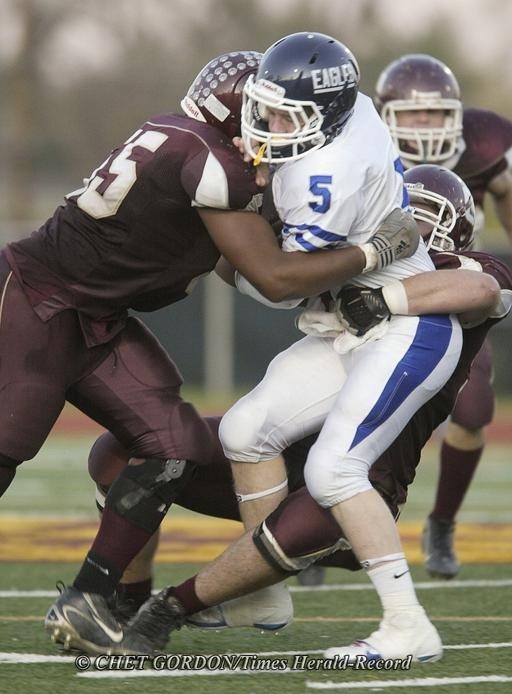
[399,163,476,251]
[180,49,265,142]
[241,30,362,165]
[372,51,463,154]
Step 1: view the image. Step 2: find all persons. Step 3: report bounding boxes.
[181,28,466,667]
[291,52,510,586]
[84,161,510,656]
[0,47,422,663]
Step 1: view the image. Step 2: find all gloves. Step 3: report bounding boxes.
[336,284,391,337]
[359,206,420,273]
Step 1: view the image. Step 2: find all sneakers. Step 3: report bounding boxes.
[421,512,460,578]
[126,584,186,650]
[45,588,162,659]
[111,579,154,624]
[187,582,294,631]
[323,629,443,665]
